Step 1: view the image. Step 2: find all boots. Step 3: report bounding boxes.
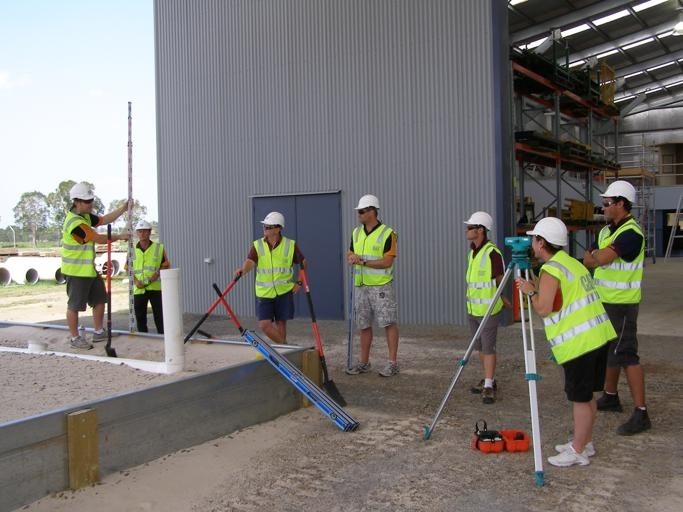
[595,390,621,411]
[616,407,651,436]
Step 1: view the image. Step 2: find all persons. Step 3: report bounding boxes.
[124,220,170,334]
[581,180,652,437]
[343,194,400,381]
[462,211,512,404]
[231,212,307,344]
[58,183,119,351]
[514,217,619,467]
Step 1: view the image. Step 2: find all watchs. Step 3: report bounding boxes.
[296,281,302,285]
[528,290,537,302]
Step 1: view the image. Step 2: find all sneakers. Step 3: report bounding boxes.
[376,362,399,376]
[481,384,495,403]
[70,336,93,350]
[547,445,589,467]
[345,359,372,374]
[555,441,595,456]
[471,377,496,393]
[92,328,118,342]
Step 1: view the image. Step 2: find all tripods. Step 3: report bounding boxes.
[422,255,599,486]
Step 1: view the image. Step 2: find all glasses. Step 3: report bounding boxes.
[603,201,616,207]
[262,225,278,230]
[357,208,373,214]
[467,225,480,230]
[79,199,93,204]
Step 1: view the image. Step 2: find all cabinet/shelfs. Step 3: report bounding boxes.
[512,63,560,234]
[587,101,618,254]
[558,84,590,261]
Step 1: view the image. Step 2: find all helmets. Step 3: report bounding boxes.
[599,180,637,204]
[353,194,379,210]
[462,211,492,231]
[134,219,151,230]
[260,212,284,228]
[69,183,93,200]
[525,216,568,246]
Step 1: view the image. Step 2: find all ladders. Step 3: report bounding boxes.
[663,194,683,262]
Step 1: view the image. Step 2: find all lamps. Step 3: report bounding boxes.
[670,20,682,36]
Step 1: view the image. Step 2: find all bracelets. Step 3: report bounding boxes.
[363,259,366,265]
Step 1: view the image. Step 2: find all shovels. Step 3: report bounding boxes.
[104,222,120,359]
[296,252,347,409]
